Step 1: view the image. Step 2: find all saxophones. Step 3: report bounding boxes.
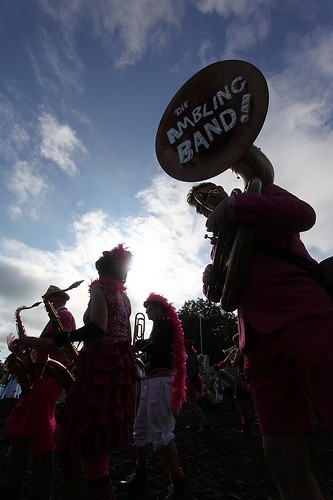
[41,280,85,389]
[5,302,42,389]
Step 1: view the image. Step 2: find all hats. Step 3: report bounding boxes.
[41,285,70,301]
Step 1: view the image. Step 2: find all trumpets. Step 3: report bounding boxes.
[132,312,145,355]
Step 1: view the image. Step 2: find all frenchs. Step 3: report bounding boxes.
[155,59,275,312]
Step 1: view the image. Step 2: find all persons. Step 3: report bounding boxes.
[53,244,134,500]
[135,333,249,431]
[0,280,84,486]
[117,293,193,490]
[188,177,332,500]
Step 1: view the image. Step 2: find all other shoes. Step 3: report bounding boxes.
[148,478,174,500]
[113,473,147,491]
